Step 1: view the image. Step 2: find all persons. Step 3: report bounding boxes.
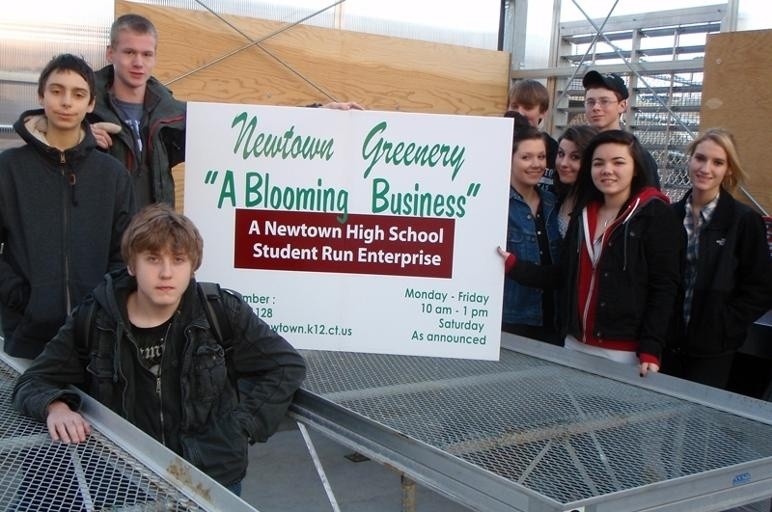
[493,127,690,504]
[10,202,307,511]
[88,12,367,214]
[550,125,602,250]
[503,78,560,192]
[499,125,570,347]
[660,129,771,478]
[1,51,140,512]
[581,69,661,197]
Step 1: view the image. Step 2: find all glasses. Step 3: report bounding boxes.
[585,100,616,108]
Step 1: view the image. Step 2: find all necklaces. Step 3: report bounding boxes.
[600,214,615,230]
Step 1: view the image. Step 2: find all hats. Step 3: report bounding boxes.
[583,70,628,99]
